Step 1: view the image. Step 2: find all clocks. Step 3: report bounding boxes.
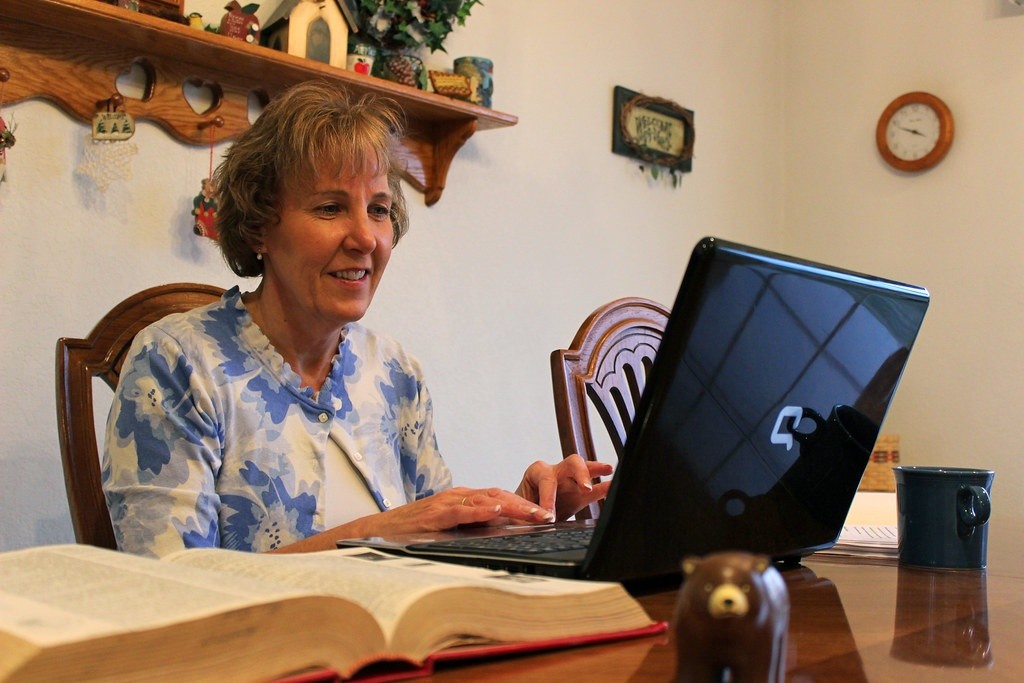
[878,91,951,171]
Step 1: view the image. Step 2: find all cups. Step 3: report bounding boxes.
[454,57,493,108]
[893,464,996,570]
[346,54,376,76]
[888,571,993,668]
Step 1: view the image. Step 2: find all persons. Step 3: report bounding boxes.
[103,84,614,557]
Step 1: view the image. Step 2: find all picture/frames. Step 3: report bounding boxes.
[612,87,693,175]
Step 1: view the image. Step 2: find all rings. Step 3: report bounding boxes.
[461,496,467,504]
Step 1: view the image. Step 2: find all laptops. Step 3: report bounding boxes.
[333,234,932,600]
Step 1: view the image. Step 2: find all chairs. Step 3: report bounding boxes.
[550,298,672,521]
[53,280,227,552]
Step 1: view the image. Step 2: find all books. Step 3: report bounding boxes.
[0,542,667,683]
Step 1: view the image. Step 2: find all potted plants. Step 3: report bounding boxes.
[347,0,480,92]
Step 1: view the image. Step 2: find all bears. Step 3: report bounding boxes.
[674,551,790,683]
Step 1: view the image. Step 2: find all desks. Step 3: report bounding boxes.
[261,511,1024,683]
